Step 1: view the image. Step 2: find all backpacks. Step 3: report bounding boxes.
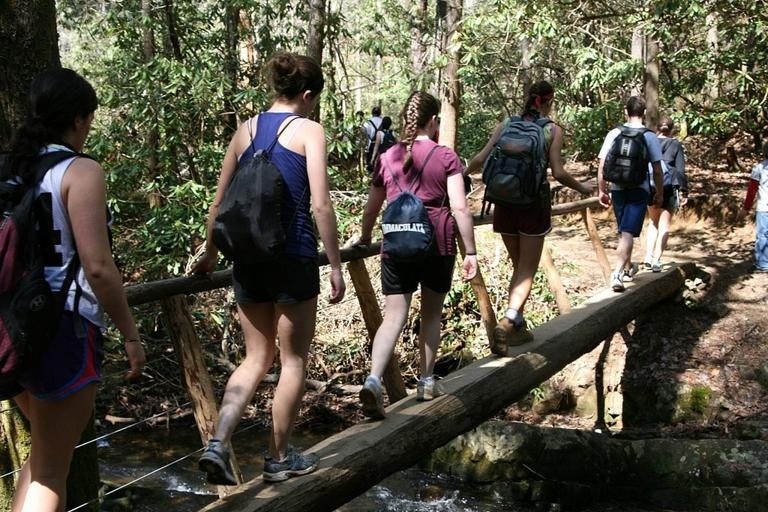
[0,149,115,400]
[602,126,655,187]
[211,116,310,267]
[380,146,447,262]
[367,120,378,158]
[647,139,673,197]
[378,130,396,153]
[479,116,554,219]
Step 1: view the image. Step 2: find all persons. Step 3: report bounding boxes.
[0,68,145,512]
[643,113,688,275]
[738,138,768,273]
[339,90,479,421]
[596,94,664,293]
[465,80,598,358]
[346,106,398,174]
[187,51,346,485]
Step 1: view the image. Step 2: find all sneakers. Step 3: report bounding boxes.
[611,258,663,292]
[489,317,515,355]
[417,375,447,400]
[359,375,387,419]
[198,439,237,486]
[747,264,767,273]
[263,444,320,483]
[508,319,533,347]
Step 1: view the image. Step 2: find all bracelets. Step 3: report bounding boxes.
[466,252,477,256]
[124,337,141,343]
